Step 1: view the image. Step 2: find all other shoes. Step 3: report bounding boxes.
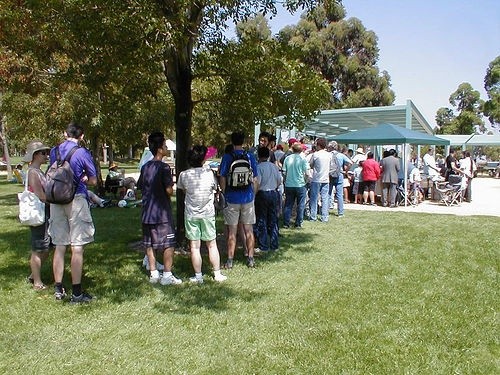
[32,284,48,290]
[100,198,112,208]
[160,276,182,285]
[54,288,67,301]
[70,291,93,304]
[190,276,203,284]
[214,276,227,282]
[143,256,164,270]
[150,274,163,284]
[222,198,398,269]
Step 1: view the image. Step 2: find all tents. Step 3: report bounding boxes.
[327,123,450,207]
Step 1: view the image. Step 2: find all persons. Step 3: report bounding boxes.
[139,129,404,284]
[109,163,137,189]
[20,142,51,291]
[135,131,182,285]
[423,148,477,201]
[16,163,112,207]
[47,123,97,302]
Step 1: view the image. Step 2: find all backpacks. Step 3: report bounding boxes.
[227,153,253,189]
[44,147,86,203]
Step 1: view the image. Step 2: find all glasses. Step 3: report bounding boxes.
[35,152,50,156]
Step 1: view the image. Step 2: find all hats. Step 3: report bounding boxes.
[109,164,117,170]
[21,143,51,162]
[355,147,365,154]
[289,138,297,144]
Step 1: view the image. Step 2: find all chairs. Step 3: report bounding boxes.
[435,175,463,207]
[397,182,425,207]
[104,175,126,200]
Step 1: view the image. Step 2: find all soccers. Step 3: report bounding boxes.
[118,200,127,208]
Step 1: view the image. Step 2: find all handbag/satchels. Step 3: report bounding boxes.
[17,191,46,227]
